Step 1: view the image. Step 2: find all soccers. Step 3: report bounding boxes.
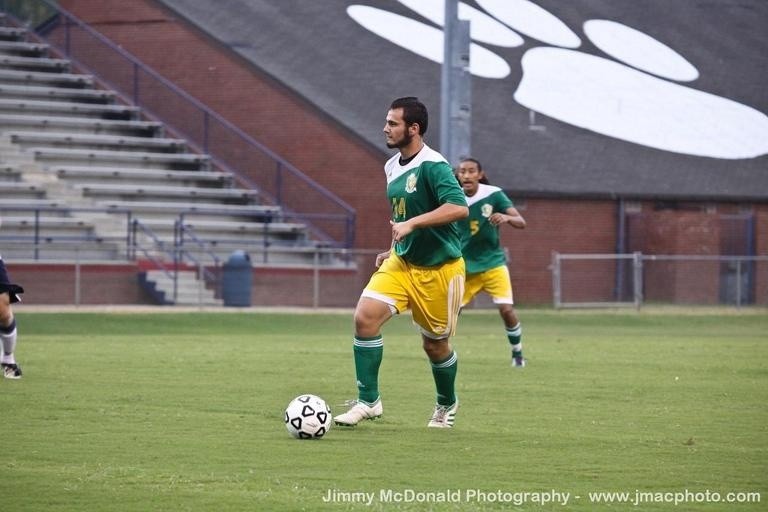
[285,394,331,439]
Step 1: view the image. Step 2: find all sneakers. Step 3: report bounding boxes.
[332,395,384,427]
[513,356,525,368]
[1,361,22,379]
[428,394,459,428]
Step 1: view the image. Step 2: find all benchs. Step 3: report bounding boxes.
[0,11,333,268]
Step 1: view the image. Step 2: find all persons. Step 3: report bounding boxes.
[454,158,527,367]
[333,94,471,429]
[0,257,25,381]
